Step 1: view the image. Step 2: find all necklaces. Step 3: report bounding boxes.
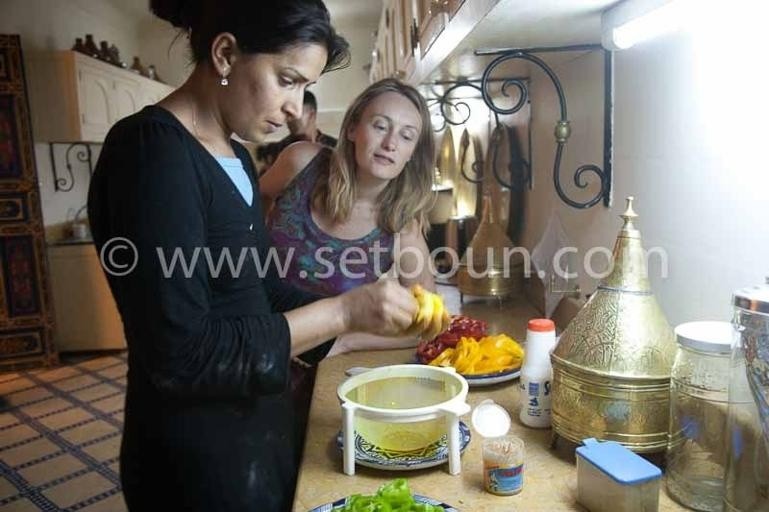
[182,90,220,153]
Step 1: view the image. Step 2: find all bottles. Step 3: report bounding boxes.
[519,318,555,428]
[72,32,165,83]
[720,277,769,511]
[664,320,746,508]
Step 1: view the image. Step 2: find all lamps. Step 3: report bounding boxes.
[598,0,702,52]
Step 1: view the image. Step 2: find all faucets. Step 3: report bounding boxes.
[68,204,88,240]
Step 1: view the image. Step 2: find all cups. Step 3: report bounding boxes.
[72,224,86,239]
[472,397,528,497]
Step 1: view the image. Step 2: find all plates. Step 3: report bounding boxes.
[307,493,460,512]
[337,420,469,470]
[412,349,526,387]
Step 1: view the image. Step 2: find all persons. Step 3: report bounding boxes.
[269,91,339,168]
[254,141,275,176]
[258,75,441,368]
[88,2,418,511]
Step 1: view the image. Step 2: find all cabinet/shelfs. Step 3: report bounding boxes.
[371,0,617,101]
[23,49,177,144]
